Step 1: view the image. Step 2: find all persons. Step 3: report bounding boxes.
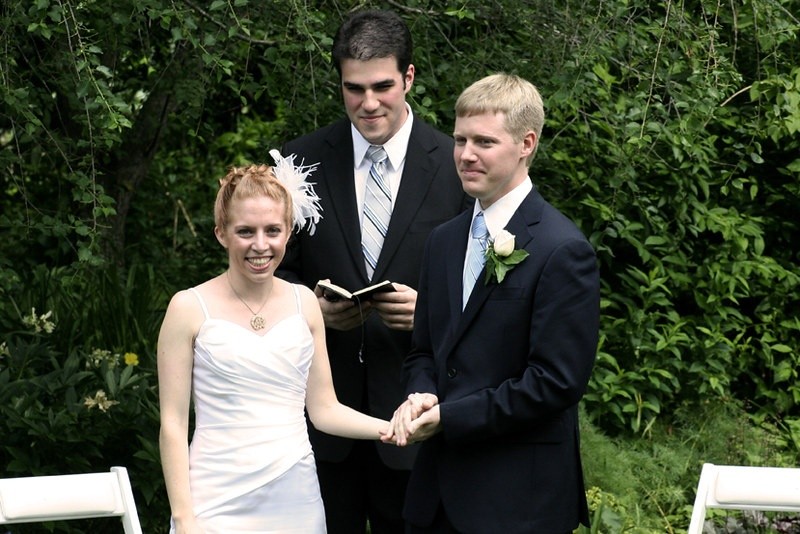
[282,7,476,534]
[155,152,420,534]
[379,72,599,534]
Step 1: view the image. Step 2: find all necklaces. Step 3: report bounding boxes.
[226,268,274,331]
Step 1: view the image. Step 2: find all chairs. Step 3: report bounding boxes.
[688,462,800,534]
[0,466,142,534]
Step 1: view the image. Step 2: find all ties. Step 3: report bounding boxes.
[360,145,392,285]
[462,213,490,313]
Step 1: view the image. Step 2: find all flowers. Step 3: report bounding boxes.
[269,149,323,235]
[479,229,529,285]
